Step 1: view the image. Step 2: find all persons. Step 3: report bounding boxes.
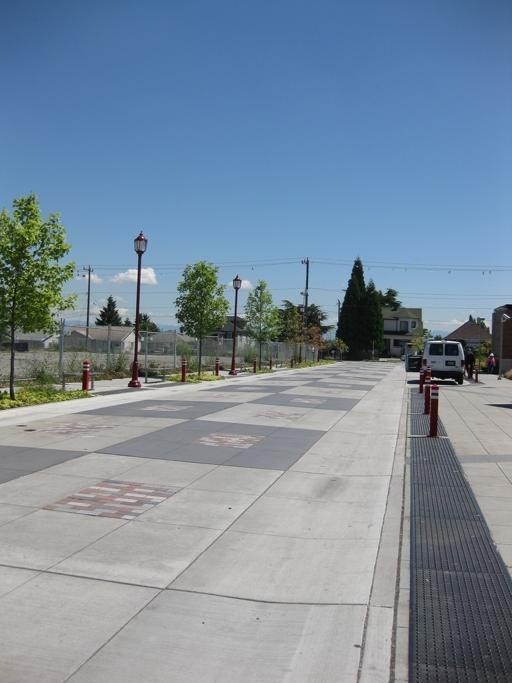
[465,347,475,379]
[486,352,497,373]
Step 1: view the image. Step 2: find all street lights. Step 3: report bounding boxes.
[228,272,242,376]
[127,229,148,388]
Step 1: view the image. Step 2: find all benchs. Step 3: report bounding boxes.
[61,371,106,392]
[137,366,177,383]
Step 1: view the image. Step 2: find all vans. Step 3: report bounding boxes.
[404,339,466,384]
